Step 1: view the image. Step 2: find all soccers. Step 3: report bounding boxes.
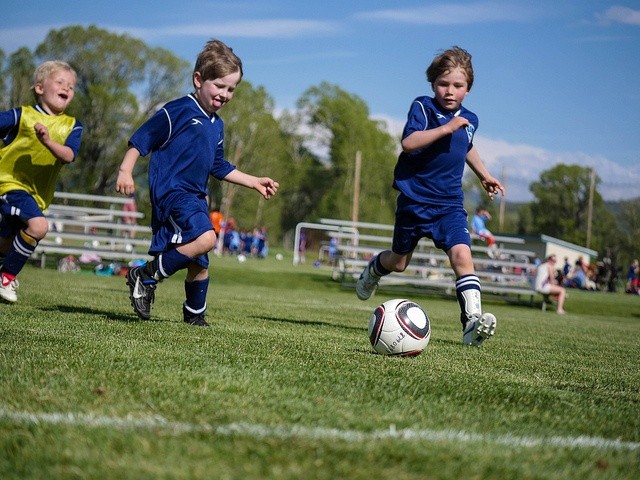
[368,299,431,357]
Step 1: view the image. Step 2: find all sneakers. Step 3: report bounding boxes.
[0,273,20,302]
[463,313,497,346]
[184,316,211,326]
[125,262,158,320]
[356,256,380,300]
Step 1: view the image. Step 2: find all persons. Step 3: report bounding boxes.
[626,260,640,294]
[533,255,567,315]
[300,232,306,263]
[563,257,570,278]
[206,206,271,260]
[574,257,587,272]
[470,207,500,258]
[115,39,280,327]
[330,238,337,264]
[551,270,563,301]
[122,192,138,238]
[355,47,505,346]
[0,60,84,303]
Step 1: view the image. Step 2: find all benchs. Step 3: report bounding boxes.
[340,254,535,287]
[44,230,152,255]
[336,244,535,272]
[319,217,525,250]
[326,230,535,264]
[34,244,154,270]
[45,216,154,234]
[53,190,134,207]
[335,266,547,311]
[47,203,143,222]
